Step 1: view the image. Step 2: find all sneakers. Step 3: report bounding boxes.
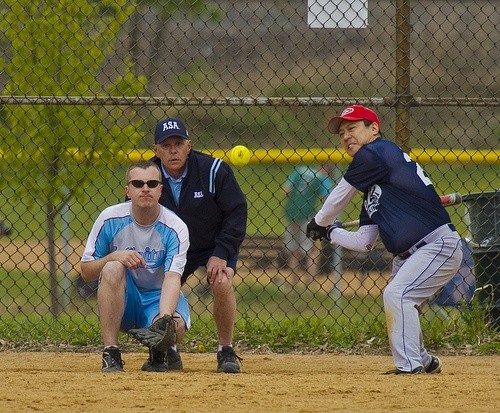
[101,344,126,373]
[381,355,442,375]
[166,347,183,369]
[141,348,167,371]
[216,346,243,373]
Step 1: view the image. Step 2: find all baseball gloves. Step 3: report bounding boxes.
[128,316,177,351]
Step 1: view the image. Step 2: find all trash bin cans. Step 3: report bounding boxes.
[460,190,500,334]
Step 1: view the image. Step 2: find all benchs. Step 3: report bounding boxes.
[239,235,395,268]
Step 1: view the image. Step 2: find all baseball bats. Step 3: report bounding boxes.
[308,192,462,238]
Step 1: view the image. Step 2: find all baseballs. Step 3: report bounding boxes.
[230,145,250,168]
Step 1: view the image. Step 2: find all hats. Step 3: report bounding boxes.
[154,116,191,145]
[328,105,380,134]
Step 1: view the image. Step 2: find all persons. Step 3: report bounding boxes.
[306,106,462,375]
[124,119,248,373]
[81,160,191,374]
[284,163,332,289]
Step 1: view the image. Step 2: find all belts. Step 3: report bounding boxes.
[398,223,457,260]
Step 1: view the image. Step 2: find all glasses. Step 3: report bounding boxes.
[127,179,163,188]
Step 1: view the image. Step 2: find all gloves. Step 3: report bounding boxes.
[305,217,343,242]
